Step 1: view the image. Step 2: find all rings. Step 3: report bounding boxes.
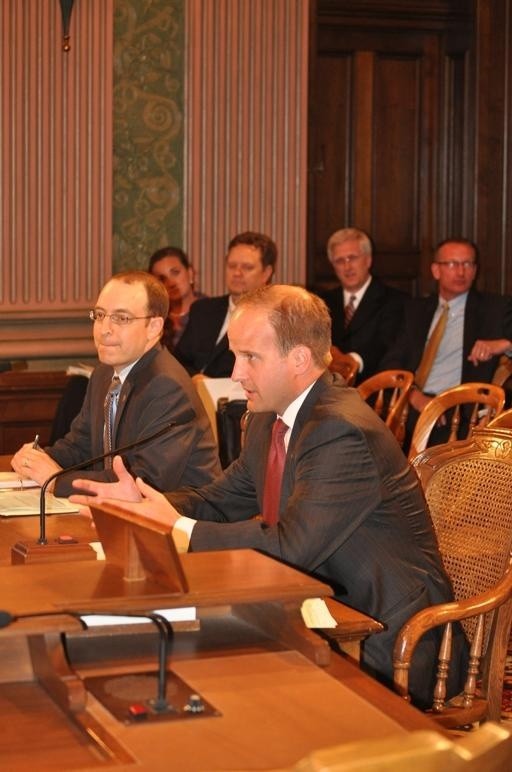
[24,457,30,467]
[478,351,486,356]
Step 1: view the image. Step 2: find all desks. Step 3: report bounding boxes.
[0,453,454,772]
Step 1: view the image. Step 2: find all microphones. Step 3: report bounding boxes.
[0,609,173,711]
[38,407,198,544]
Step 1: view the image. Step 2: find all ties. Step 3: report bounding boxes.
[412,304,449,390]
[104,378,121,470]
[344,295,355,328]
[262,419,289,528]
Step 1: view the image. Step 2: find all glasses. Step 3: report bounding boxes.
[87,309,155,327]
[433,260,476,270]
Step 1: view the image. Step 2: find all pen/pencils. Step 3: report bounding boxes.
[32,434,39,448]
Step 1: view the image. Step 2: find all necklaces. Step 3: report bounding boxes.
[164,309,189,317]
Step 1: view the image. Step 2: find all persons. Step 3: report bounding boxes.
[11,268,222,505]
[384,237,511,451]
[310,227,405,382]
[146,246,212,356]
[66,282,470,710]
[169,230,277,378]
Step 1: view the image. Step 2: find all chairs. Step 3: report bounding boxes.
[485,355,512,416]
[293,717,512,772]
[329,344,360,387]
[392,427,512,730]
[239,369,415,450]
[408,383,506,463]
